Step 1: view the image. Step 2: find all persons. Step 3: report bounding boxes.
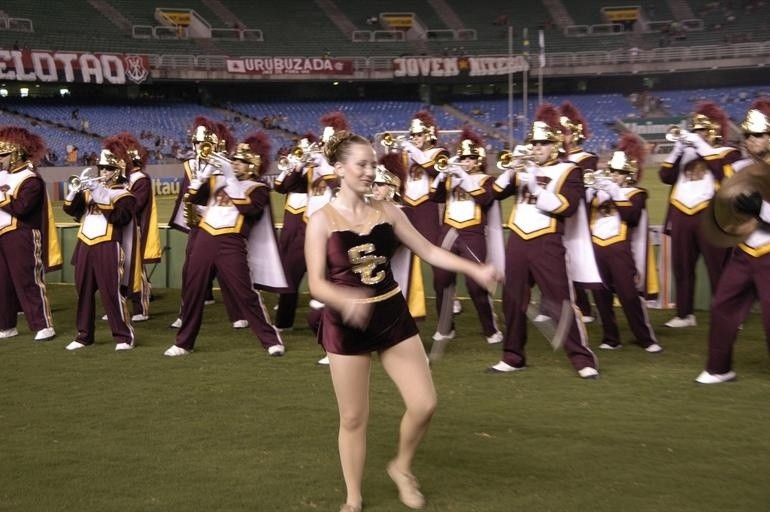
[302,129,508,511]
[1,95,769,385]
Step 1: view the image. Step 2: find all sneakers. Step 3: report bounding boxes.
[0,299,294,356]
[338,499,363,512]
[692,364,738,386]
[449,299,698,353]
[384,457,426,511]
[491,359,601,379]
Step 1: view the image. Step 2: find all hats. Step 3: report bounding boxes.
[609,150,640,176]
[98,147,141,169]
[529,115,583,144]
[0,139,25,157]
[738,109,770,137]
[408,118,432,136]
[295,125,335,150]
[232,142,262,166]
[191,125,219,145]
[685,109,721,133]
[371,164,403,189]
[456,139,480,158]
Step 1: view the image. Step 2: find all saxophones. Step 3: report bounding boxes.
[183,161,201,227]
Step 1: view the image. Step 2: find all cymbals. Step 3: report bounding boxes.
[700,159,770,249]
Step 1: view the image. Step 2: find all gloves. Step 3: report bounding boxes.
[583,169,627,203]
[493,143,545,196]
[306,152,333,171]
[430,166,472,190]
[399,140,427,162]
[193,154,239,186]
[665,131,715,164]
[65,175,108,204]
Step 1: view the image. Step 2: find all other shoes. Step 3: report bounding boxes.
[317,355,329,366]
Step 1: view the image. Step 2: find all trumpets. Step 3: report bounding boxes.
[199,143,239,173]
[496,148,537,167]
[294,148,324,159]
[435,155,467,171]
[278,156,295,171]
[382,133,416,149]
[666,125,693,148]
[583,168,615,189]
[67,176,108,194]
